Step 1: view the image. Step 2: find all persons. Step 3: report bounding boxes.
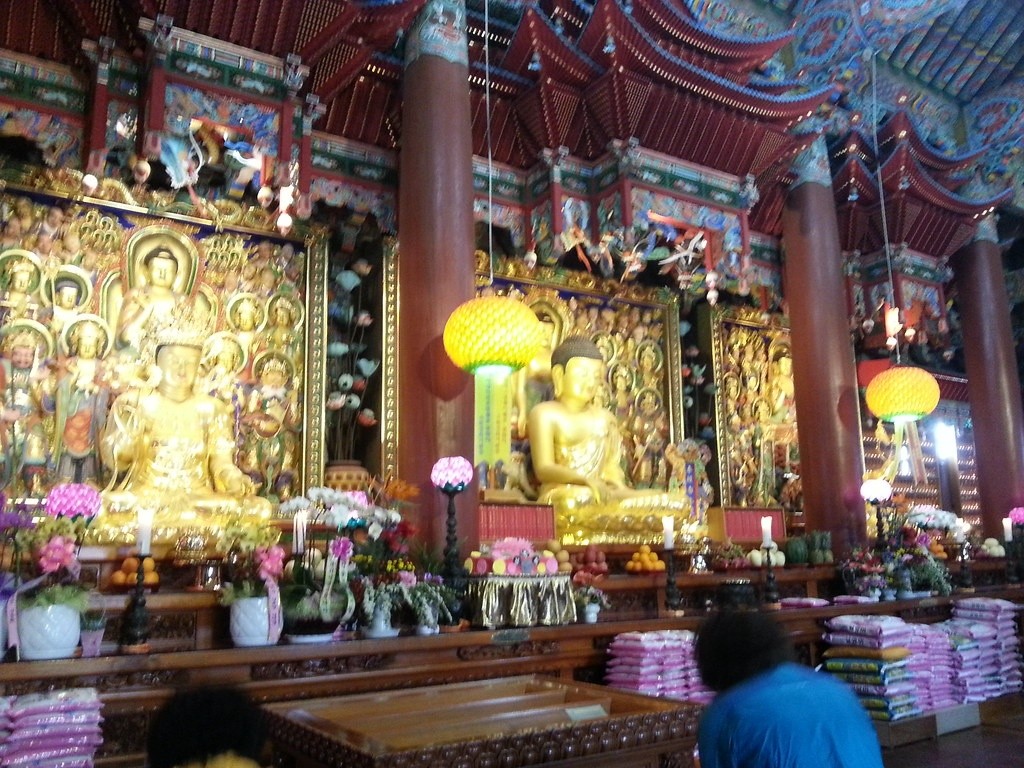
[528,335,654,509]
[54,324,103,480]
[3,197,97,287]
[0,330,43,493]
[0,258,37,320]
[92,320,273,525]
[695,610,884,768]
[237,301,293,344]
[207,343,301,502]
[45,282,80,332]
[117,246,188,344]
[770,350,796,415]
[219,240,302,298]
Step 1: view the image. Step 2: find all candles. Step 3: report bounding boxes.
[761,516,772,547]
[138,510,154,553]
[292,510,308,554]
[662,516,674,548]
[1002,518,1012,542]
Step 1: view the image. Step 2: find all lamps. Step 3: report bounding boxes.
[860,479,892,552]
[1009,507,1024,535]
[431,457,473,576]
[956,518,964,543]
[46,483,100,516]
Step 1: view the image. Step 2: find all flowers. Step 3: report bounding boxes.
[0,491,86,585]
[222,486,448,585]
[573,572,612,608]
[840,495,929,593]
[324,257,380,460]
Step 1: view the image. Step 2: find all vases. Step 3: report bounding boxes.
[230,597,283,647]
[324,460,371,491]
[17,603,82,661]
[418,606,440,636]
[882,588,896,600]
[584,604,600,623]
[0,571,23,661]
[284,593,345,643]
[866,587,881,602]
[898,577,915,599]
[364,598,398,638]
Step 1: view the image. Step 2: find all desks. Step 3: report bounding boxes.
[0,549,1016,667]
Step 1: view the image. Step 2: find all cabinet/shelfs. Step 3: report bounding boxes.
[0,543,1024,768]
